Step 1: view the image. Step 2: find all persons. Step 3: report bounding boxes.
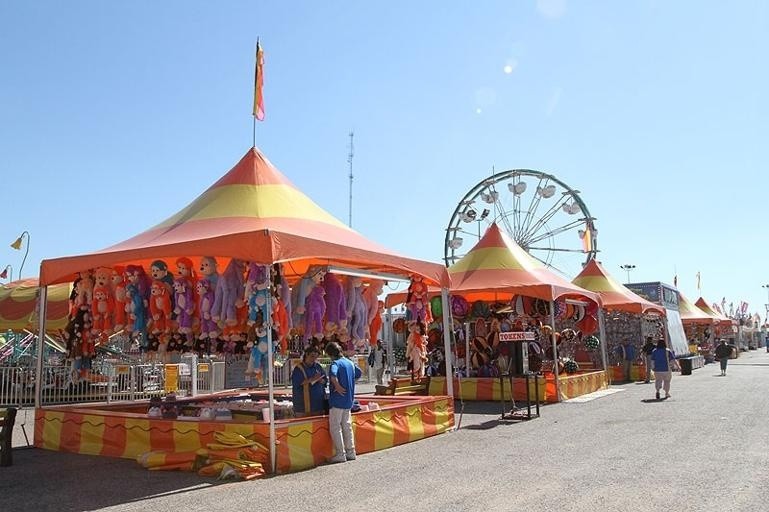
[292,348,328,418]
[367,338,387,383]
[620,339,634,382]
[716,341,732,376]
[641,337,658,383]
[651,340,681,400]
[688,339,698,355]
[324,342,362,463]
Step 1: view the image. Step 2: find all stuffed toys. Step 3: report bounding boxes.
[290,264,385,356]
[64,258,196,380]
[196,254,293,374]
[404,271,433,383]
[63,255,437,388]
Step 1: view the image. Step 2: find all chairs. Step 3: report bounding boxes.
[0,407,17,465]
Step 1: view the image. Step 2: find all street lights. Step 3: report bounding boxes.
[619,264,637,283]
[762,283,769,302]
[1,264,13,284]
[10,231,31,280]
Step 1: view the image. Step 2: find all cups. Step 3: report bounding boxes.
[262,407,270,422]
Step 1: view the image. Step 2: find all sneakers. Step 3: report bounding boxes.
[656,392,661,399]
[329,454,347,463]
[665,394,671,397]
[347,452,356,460]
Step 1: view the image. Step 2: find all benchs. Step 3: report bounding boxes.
[373,375,430,394]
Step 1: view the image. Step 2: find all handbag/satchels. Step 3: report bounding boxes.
[666,348,681,371]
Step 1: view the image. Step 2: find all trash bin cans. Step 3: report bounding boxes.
[680,358,692,375]
